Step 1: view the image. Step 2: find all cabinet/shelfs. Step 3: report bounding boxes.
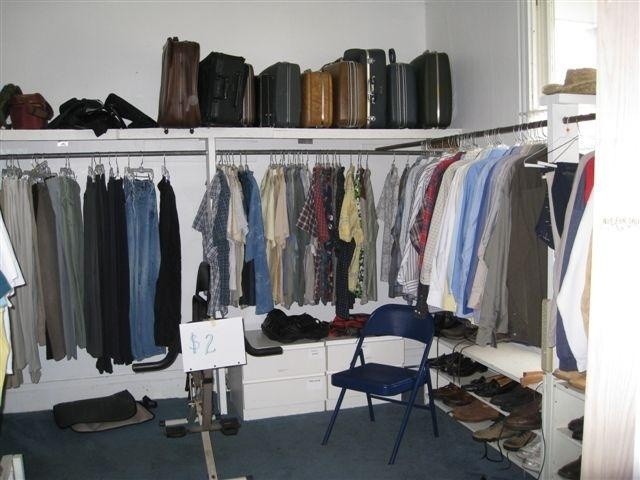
[548,380,585,480]
[427,332,543,480]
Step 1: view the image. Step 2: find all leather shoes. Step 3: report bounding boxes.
[425,311,586,480]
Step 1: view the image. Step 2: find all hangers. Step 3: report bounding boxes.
[1,152,171,185]
[417,112,593,171]
[216,148,411,175]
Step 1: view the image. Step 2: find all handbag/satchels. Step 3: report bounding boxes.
[0,84,158,137]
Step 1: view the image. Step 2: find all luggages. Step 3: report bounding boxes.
[158,37,451,128]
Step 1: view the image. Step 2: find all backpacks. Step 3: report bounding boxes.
[262,309,371,343]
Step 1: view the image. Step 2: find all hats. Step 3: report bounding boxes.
[543,68,596,95]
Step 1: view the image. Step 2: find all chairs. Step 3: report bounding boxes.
[321,303,439,466]
